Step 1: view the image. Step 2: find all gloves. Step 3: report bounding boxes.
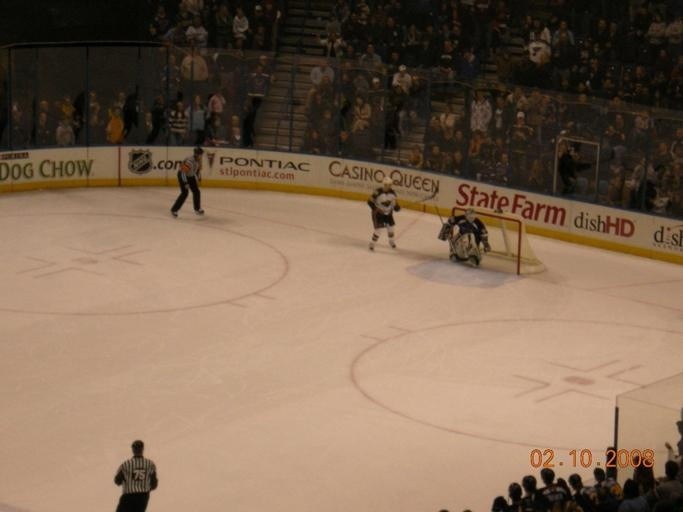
[375,208,384,216]
[394,205,401,212]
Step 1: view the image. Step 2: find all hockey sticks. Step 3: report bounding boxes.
[436,205,469,261]
[399,186,439,207]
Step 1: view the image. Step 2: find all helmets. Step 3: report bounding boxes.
[382,176,393,190]
[465,209,475,223]
[132,440,144,453]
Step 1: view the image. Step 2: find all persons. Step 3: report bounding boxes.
[112,440,157,512]
[367,178,401,250]
[1,2,286,148]
[297,1,683,221]
[490,408,683,512]
[439,206,490,267]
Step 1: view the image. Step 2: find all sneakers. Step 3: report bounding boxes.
[171,207,205,217]
[368,240,396,251]
